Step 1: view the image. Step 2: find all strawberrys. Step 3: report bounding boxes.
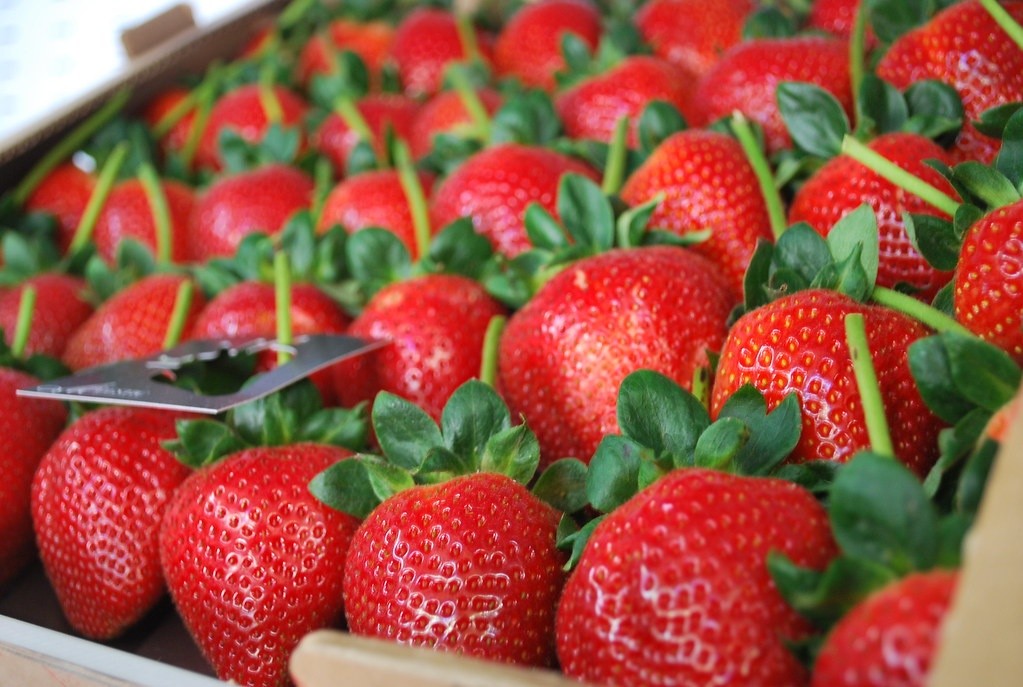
[0,0,1023,687]
